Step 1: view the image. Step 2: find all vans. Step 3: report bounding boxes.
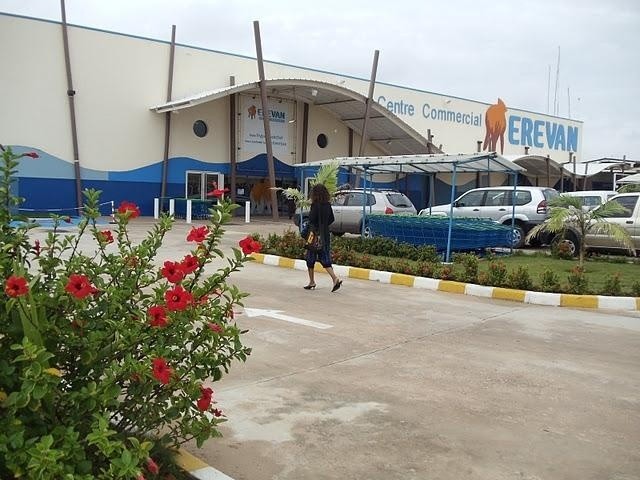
[557,191,619,218]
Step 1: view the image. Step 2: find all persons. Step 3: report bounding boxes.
[304,181,342,292]
[287,195,296,219]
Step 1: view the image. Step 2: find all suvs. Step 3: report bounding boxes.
[418,186,564,250]
[290,184,420,240]
[538,192,639,260]
